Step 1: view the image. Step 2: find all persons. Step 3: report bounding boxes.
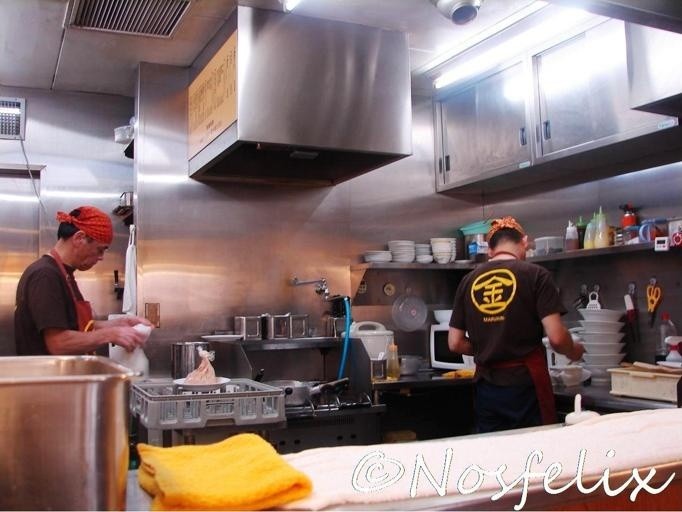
[447,216,587,432]
[12,207,155,356]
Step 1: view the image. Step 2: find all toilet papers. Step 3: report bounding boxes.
[369,350,387,380]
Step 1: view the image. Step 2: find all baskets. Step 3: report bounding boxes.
[129,379,287,431]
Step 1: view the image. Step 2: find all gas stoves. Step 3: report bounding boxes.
[285,390,387,420]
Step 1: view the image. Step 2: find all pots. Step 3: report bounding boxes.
[169,341,210,380]
[261,377,350,408]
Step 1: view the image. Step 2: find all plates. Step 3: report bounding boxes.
[200,334,243,342]
[173,377,232,392]
[455,259,473,263]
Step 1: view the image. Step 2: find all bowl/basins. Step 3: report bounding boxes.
[433,309,453,325]
[398,354,423,377]
[459,218,493,237]
[575,308,627,382]
[548,365,583,386]
[363,237,457,264]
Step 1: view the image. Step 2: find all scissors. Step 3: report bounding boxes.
[645,285,662,327]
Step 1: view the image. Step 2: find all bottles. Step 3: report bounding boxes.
[653,311,677,365]
[564,205,609,251]
[386,342,400,382]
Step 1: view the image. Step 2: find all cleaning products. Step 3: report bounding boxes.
[619,202,641,240]
[663,335,682,362]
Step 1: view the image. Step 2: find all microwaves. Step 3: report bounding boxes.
[430,324,476,370]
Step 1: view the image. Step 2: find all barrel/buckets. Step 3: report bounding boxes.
[0,355,144,512]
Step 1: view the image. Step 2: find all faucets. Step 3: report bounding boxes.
[291,274,330,299]
